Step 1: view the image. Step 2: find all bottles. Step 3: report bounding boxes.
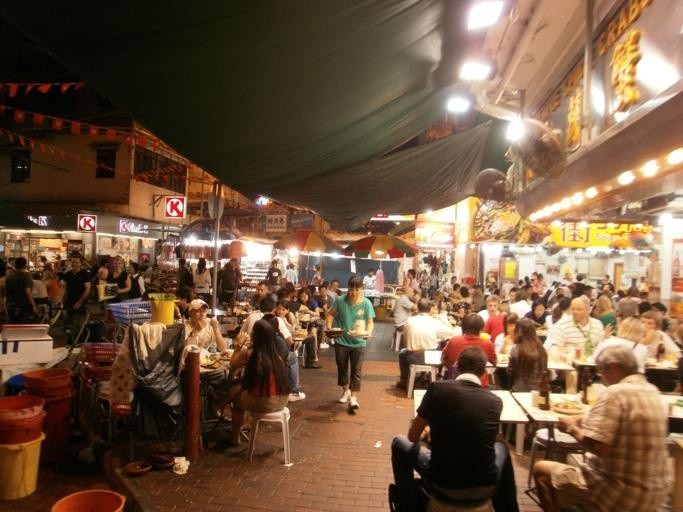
[581,367,592,404]
[539,370,550,410]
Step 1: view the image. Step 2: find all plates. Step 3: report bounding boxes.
[346,331,374,338]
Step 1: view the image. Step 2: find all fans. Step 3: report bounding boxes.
[512,118,565,180]
[474,168,515,210]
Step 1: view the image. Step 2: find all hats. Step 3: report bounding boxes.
[188,298,210,311]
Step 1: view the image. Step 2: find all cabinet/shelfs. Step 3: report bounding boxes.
[241,265,269,283]
[4,234,61,273]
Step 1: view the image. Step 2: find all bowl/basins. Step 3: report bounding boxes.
[324,329,344,338]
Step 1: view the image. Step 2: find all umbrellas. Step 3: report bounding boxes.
[340,235,418,277]
[179,219,244,240]
[273,230,344,279]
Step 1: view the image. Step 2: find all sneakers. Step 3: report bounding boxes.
[348,396,360,409]
[339,389,351,403]
[288,392,305,402]
[319,342,330,349]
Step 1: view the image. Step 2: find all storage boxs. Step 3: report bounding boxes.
[0,324,53,365]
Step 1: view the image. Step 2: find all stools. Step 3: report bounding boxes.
[524,428,588,492]
[505,422,542,457]
[391,330,403,351]
[247,407,292,467]
[426,497,496,512]
[406,364,436,399]
[37,304,48,323]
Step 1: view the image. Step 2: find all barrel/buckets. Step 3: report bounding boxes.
[0,396,46,418]
[0,432,47,501]
[0,412,47,444]
[23,367,74,385]
[51,489,127,512]
[19,391,74,465]
[23,383,74,397]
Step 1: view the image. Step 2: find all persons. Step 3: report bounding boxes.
[362,268,377,303]
[1,250,152,346]
[194,258,211,299]
[183,298,227,419]
[534,347,675,511]
[218,257,338,451]
[392,346,519,512]
[326,277,376,409]
[176,258,194,301]
[390,271,683,392]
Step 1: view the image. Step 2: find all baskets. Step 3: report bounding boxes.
[79,342,113,363]
[79,364,113,391]
[108,301,152,324]
